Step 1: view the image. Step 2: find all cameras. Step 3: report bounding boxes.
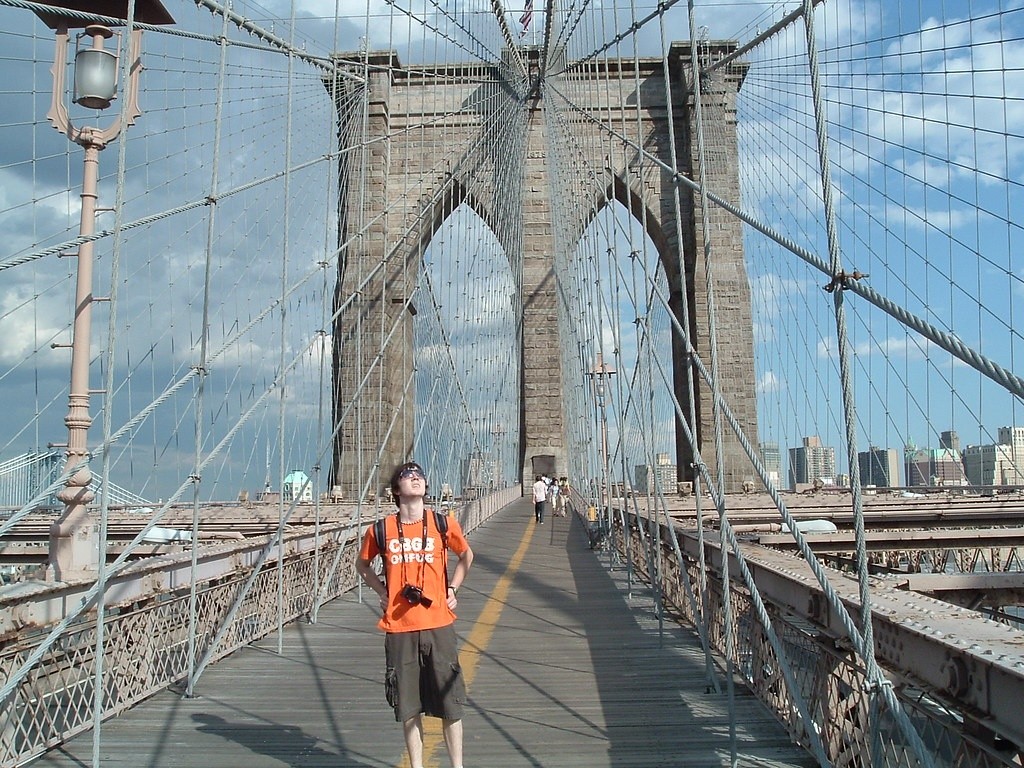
[400,584,432,609]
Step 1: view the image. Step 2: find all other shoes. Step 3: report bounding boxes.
[540,521,544,525]
[562,515,564,517]
[554,513,556,515]
[556,514,558,517]
[536,519,539,524]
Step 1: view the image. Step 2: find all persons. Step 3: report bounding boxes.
[354,463,473,768]
[532,474,571,524]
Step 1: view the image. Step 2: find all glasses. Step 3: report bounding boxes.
[397,469,426,481]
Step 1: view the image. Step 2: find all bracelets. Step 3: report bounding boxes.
[448,586,458,596]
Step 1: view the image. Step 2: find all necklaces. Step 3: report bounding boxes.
[402,518,424,525]
